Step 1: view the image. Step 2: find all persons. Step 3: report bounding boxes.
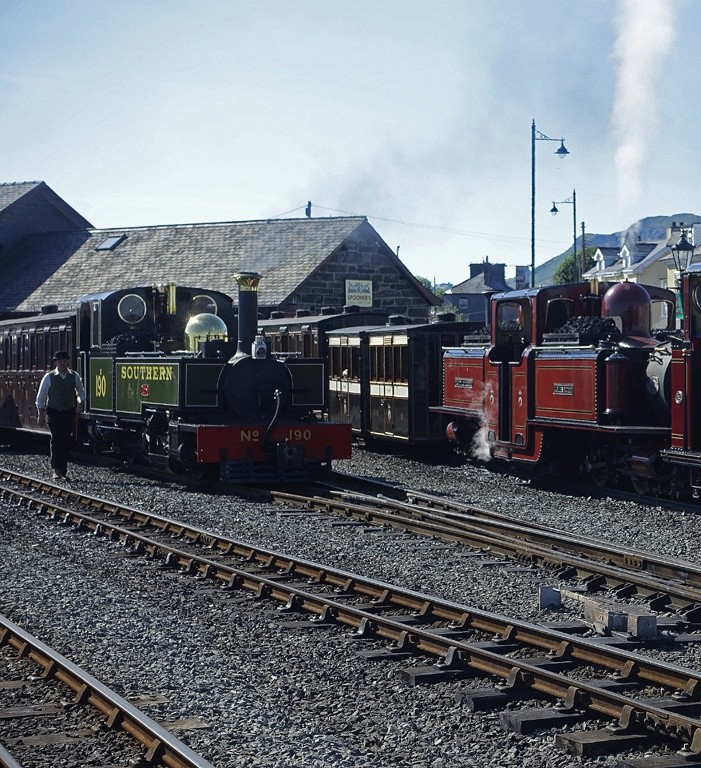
[34,350,87,481]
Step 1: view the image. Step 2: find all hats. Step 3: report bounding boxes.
[52,351,69,360]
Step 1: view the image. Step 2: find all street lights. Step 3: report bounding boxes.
[550,188,578,282]
[532,119,571,287]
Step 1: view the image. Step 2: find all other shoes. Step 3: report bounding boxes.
[52,469,59,478]
[60,471,68,479]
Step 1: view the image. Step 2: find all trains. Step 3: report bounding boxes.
[1,270,353,492]
[263,270,701,506]
